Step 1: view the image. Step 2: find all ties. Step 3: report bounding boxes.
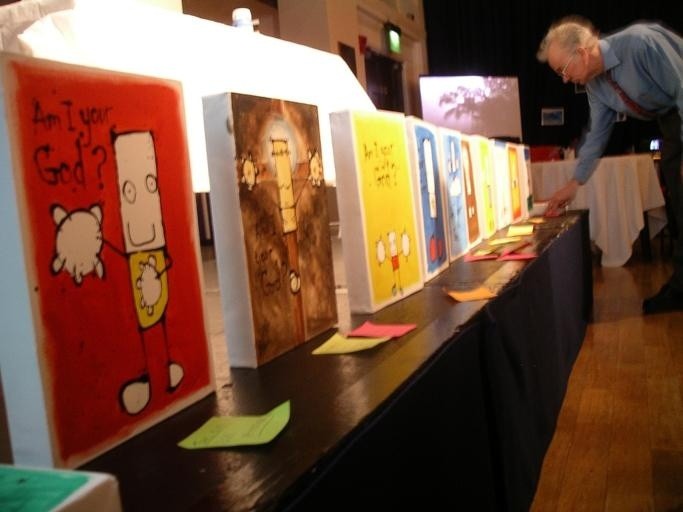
[601,68,664,120]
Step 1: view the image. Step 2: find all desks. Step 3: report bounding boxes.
[532,152,670,270]
[72,208,596,511]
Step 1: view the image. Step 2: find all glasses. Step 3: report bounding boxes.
[557,51,577,79]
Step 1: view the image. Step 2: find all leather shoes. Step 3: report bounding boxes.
[640,270,683,314]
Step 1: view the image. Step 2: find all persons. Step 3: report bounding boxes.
[537,15,683,312]
